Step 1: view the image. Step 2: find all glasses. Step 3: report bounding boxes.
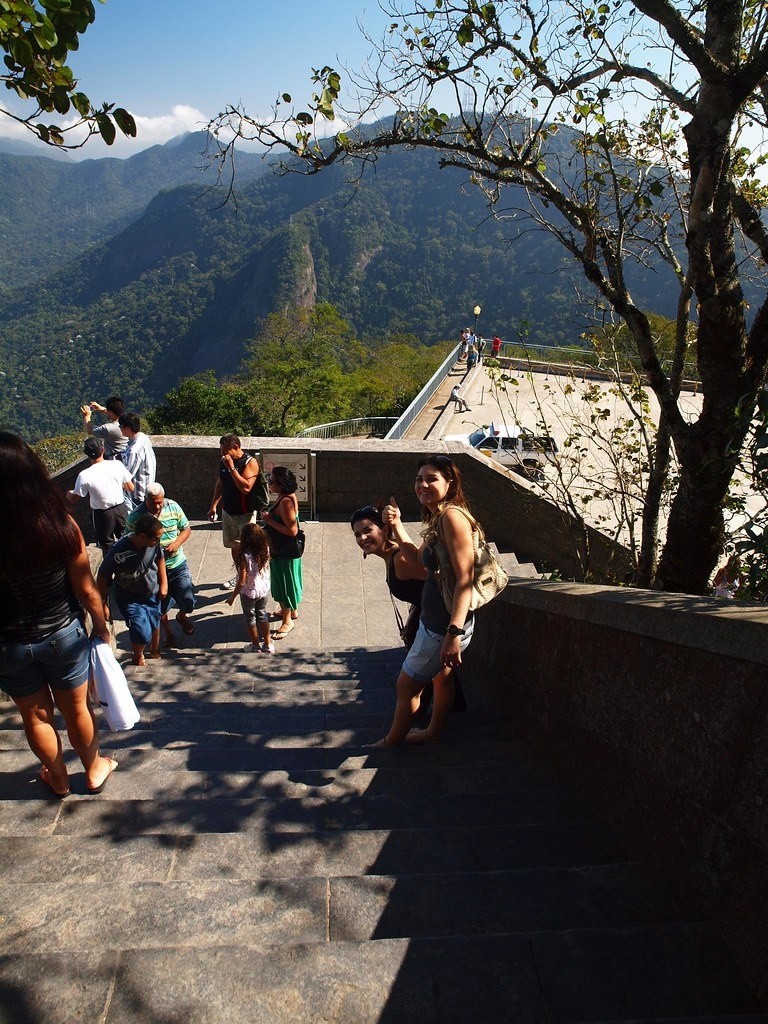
[350,506,380,523]
[269,478,272,485]
[427,456,455,481]
[119,425,123,428]
[153,538,160,546]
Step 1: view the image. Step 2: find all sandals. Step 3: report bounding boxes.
[362,738,400,750]
[403,728,444,744]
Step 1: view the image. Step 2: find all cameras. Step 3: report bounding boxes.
[90,405,95,411]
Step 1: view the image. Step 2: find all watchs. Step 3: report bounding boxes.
[228,468,238,473]
[446,625,466,638]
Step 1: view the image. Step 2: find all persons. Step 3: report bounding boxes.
[461,328,486,371]
[491,335,501,358]
[97,513,168,667]
[0,430,118,798]
[350,506,467,712]
[361,454,476,748]
[450,383,472,413]
[207,433,259,590]
[65,437,135,559]
[122,482,197,649]
[713,554,743,597]
[225,523,275,653]
[118,410,156,506]
[259,467,303,640]
[80,397,129,462]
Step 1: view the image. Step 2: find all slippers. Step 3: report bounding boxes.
[176,615,196,635]
[86,757,116,794]
[275,610,297,619]
[271,627,294,640]
[40,769,72,797]
[163,632,174,648]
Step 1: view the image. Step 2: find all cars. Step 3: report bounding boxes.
[442,425,560,482]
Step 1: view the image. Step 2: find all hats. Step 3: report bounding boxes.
[85,437,102,455]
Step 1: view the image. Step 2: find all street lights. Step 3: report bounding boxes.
[473,305,482,340]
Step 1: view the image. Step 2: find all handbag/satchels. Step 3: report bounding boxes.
[401,605,420,649]
[238,456,269,511]
[262,495,305,559]
[437,506,509,615]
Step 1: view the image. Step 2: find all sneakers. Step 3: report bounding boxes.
[223,577,237,589]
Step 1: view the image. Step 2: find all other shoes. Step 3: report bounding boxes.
[132,655,146,665]
[148,643,161,659]
[460,410,465,413]
[466,409,471,411]
[244,644,262,653]
[260,642,275,653]
